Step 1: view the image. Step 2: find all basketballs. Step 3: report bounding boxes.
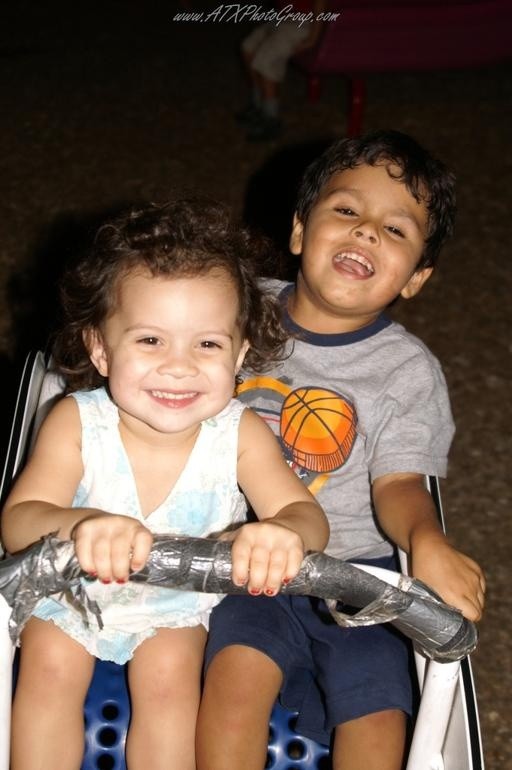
[280,387,357,472]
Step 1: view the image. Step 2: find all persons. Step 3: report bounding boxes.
[233,3,327,140]
[196,128,485,770]
[1,196,329,770]
